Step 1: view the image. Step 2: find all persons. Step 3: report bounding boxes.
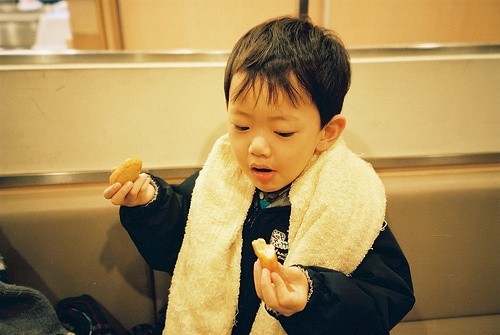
[102,15,415,335]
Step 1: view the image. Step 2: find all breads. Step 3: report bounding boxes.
[252,238,279,272]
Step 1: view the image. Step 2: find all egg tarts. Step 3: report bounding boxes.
[109,157,142,186]
